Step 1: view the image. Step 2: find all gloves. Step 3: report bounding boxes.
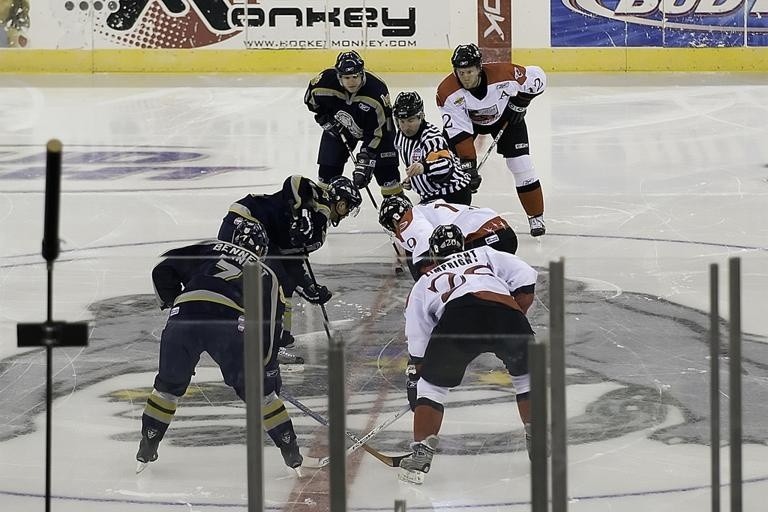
[465,167,482,193]
[289,214,316,247]
[404,376,424,412]
[266,361,283,392]
[297,274,332,306]
[314,113,343,138]
[504,97,533,122]
[353,150,379,188]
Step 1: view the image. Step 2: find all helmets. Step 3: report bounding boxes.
[231,218,269,262]
[392,90,424,118]
[335,50,366,91]
[452,43,486,68]
[328,176,362,227]
[429,223,465,264]
[378,194,413,237]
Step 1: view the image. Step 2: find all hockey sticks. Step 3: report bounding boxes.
[279,388,414,467]
[340,133,411,276]
[297,405,412,469]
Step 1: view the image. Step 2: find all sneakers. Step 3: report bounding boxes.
[529,213,545,237]
[400,434,441,474]
[136,436,160,464]
[279,437,302,468]
[277,349,303,364]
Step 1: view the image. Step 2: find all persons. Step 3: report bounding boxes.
[393,91,472,205]
[218,177,363,371]
[137,219,304,477]
[304,50,405,197]
[379,196,518,280]
[435,43,547,237]
[399,224,540,485]
